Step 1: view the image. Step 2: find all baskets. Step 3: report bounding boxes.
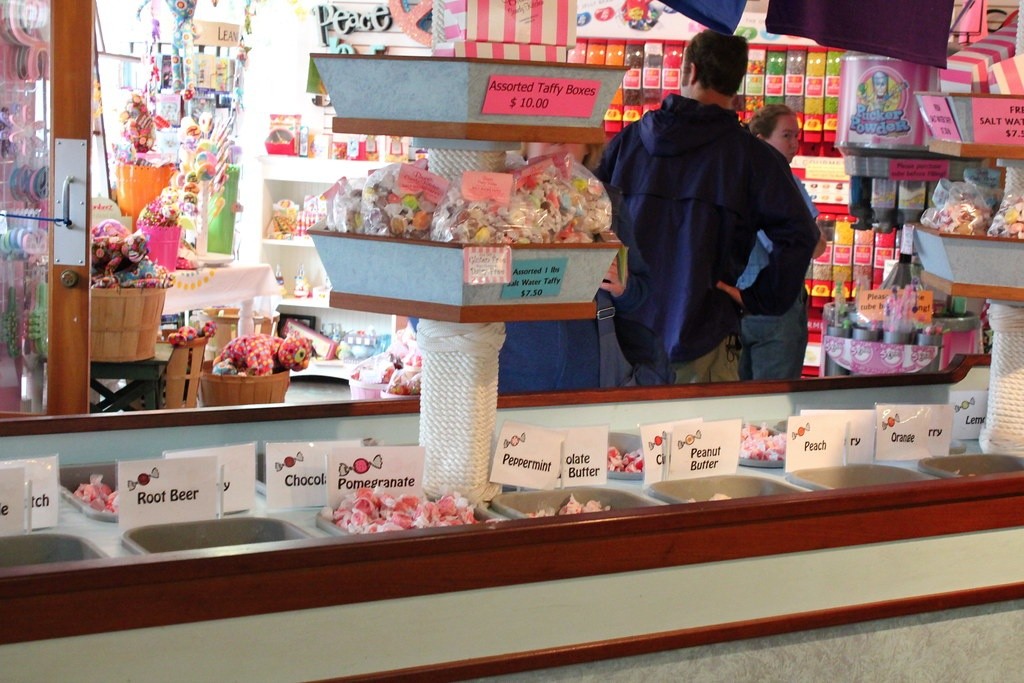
[200,360,290,407]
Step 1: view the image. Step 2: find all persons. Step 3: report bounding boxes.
[736,105,839,384]
[590,31,825,385]
[405,121,660,397]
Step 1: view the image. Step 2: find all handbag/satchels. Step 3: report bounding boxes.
[599,337,676,388]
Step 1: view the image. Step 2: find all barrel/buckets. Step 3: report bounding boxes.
[200,361,290,407]
[90,288,165,363]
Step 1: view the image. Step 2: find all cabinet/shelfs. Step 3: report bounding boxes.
[236,157,411,381]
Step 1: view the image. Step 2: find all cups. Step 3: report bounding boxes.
[137,226,182,271]
[115,164,174,234]
[207,167,240,255]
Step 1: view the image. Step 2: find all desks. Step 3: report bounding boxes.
[87,343,174,413]
[162,263,283,339]
